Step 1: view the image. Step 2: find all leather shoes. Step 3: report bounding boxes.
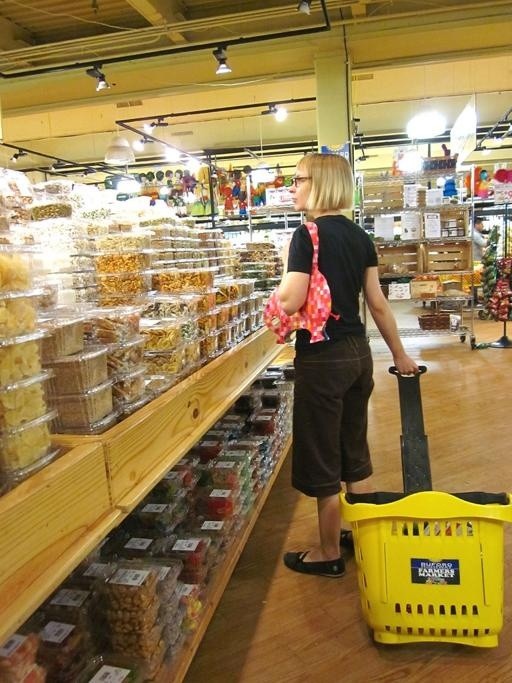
[284,550,345,579]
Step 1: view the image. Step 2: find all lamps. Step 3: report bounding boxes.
[132,114,168,152]
[261,103,288,122]
[86,62,112,90]
[212,41,232,74]
[104,123,135,163]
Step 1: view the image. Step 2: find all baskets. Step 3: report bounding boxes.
[418,312,451,330]
[340,488,512,651]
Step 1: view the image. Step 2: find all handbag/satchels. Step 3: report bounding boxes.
[263,220,342,346]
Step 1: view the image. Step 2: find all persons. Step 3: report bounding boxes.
[155,171,164,182]
[215,164,268,222]
[467,218,491,306]
[165,170,174,180]
[139,173,147,183]
[174,169,183,180]
[272,149,420,579]
[146,172,155,182]
